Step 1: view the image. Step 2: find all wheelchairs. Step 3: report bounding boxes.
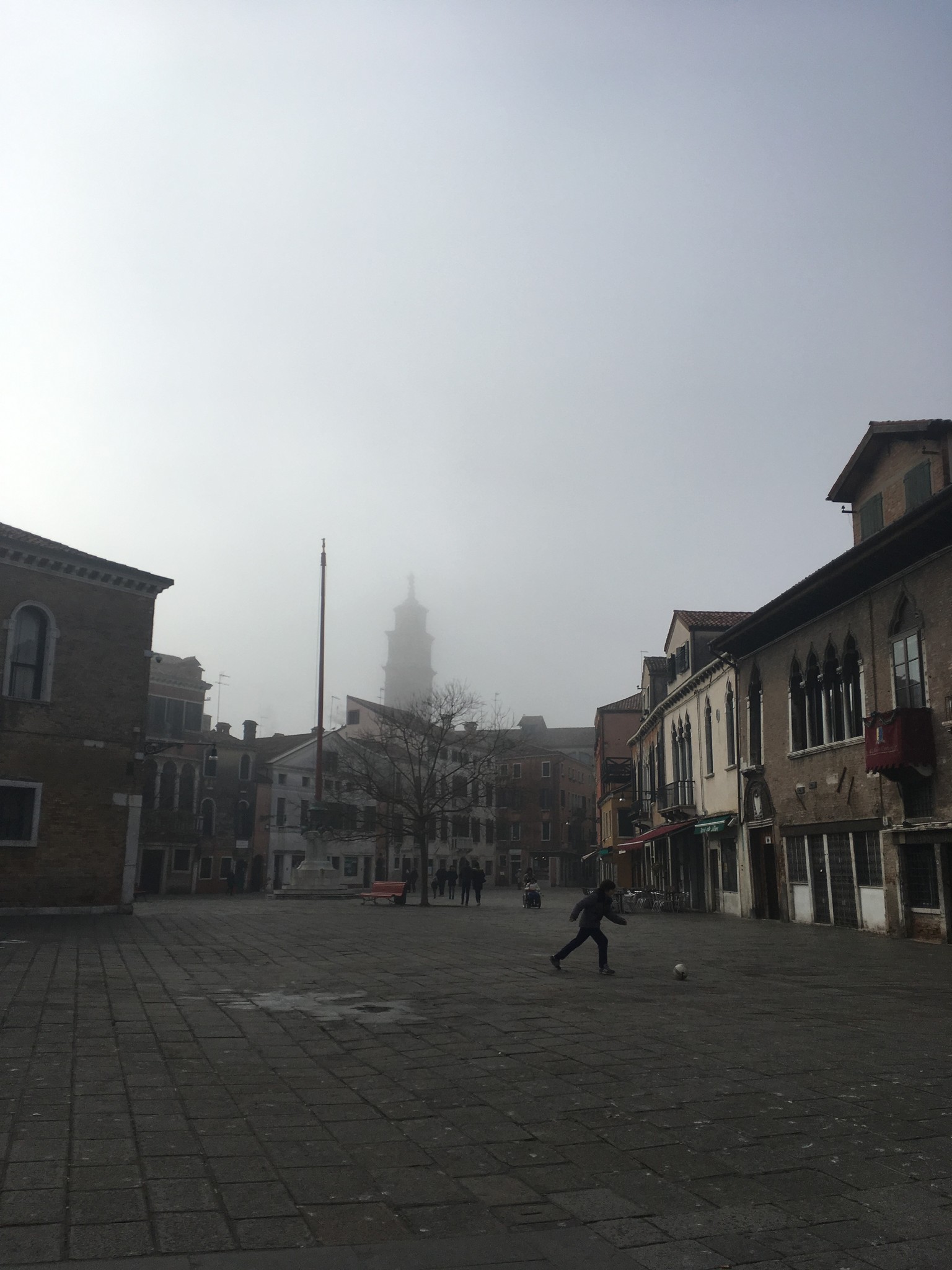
[522,889,544,909]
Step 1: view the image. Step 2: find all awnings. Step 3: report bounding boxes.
[617,820,696,851]
[694,815,731,834]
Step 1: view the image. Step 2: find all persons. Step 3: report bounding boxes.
[403,870,411,893]
[431,877,438,898]
[526,878,541,907]
[517,869,523,890]
[459,861,472,905]
[446,865,458,900]
[469,862,485,906]
[226,869,235,895]
[409,867,418,892]
[523,867,536,886]
[435,865,448,896]
[550,879,627,974]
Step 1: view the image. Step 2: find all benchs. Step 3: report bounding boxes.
[360,880,407,906]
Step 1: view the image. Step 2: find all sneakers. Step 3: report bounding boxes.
[549,955,560,970]
[599,964,615,974]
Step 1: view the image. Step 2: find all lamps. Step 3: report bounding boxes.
[566,817,598,825]
[260,815,287,822]
[620,790,656,801]
[144,740,218,761]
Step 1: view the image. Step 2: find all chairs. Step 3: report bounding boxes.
[587,889,689,913]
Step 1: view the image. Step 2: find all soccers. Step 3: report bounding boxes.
[673,964,688,979]
